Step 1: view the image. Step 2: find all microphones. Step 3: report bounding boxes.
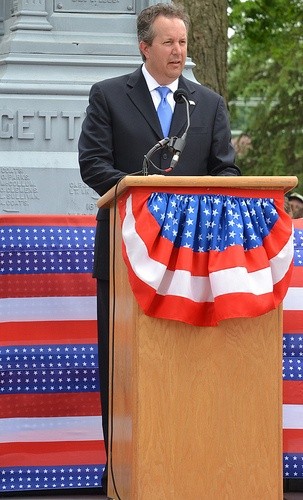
[173,89,191,154]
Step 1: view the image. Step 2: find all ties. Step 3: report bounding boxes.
[154,86,175,138]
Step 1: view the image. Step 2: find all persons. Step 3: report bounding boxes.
[237,134,253,157]
[77,3,244,498]
[288,192,303,220]
